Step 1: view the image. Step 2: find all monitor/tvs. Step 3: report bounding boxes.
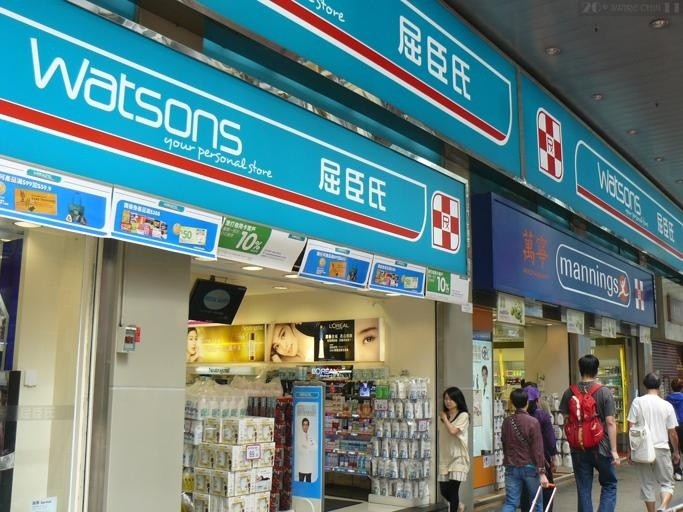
[189,278,246,325]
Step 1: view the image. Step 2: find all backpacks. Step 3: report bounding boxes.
[563,384,605,452]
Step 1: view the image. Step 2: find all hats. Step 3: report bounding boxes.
[524,384,540,401]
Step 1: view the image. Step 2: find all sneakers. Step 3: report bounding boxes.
[674,472,682,481]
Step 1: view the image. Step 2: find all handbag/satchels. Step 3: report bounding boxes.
[629,395,656,464]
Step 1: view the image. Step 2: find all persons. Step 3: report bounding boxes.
[523,385,557,512]
[437,387,470,512]
[353,319,379,362]
[498,388,551,512]
[665,377,681,484]
[476,365,491,450]
[625,372,681,512]
[267,323,314,363]
[296,416,315,483]
[558,353,621,512]
[186,327,205,363]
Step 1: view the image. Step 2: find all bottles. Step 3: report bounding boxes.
[247,333,256,360]
